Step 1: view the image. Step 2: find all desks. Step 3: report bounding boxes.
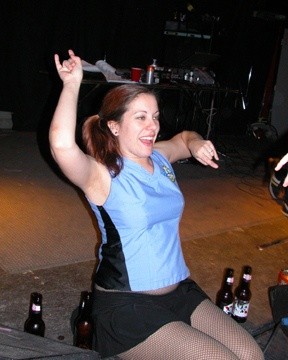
[75,69,233,163]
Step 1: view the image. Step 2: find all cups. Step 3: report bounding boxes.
[132,68,141,82]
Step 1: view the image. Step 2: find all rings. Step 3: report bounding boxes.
[209,150,213,153]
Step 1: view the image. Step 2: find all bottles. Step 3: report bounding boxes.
[216,266,252,323]
[70,291,97,350]
[24,292,45,337]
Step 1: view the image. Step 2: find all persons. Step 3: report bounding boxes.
[49,50,265,360]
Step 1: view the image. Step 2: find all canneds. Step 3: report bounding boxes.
[145,66,155,84]
[277,268,288,285]
